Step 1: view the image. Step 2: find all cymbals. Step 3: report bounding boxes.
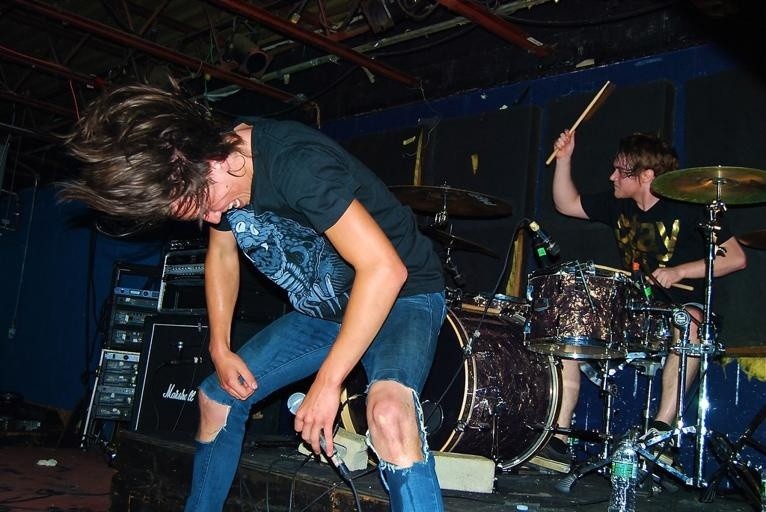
[651,166,766,204]
[737,230,766,249]
[386,185,512,219]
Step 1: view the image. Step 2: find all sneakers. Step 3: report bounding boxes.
[528,441,573,475]
[653,444,675,466]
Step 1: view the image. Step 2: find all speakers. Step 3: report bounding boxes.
[128,314,301,443]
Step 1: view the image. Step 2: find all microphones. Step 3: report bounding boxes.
[286,392,352,482]
[529,220,560,256]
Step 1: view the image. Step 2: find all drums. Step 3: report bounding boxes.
[463,289,529,320]
[340,305,562,475]
[522,259,633,360]
[626,297,677,359]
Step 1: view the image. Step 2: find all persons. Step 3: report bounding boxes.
[527,128,748,474]
[55,79,446,512]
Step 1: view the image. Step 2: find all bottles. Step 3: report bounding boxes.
[609,437,641,511]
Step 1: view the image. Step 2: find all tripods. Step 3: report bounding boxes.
[555,185,766,512]
